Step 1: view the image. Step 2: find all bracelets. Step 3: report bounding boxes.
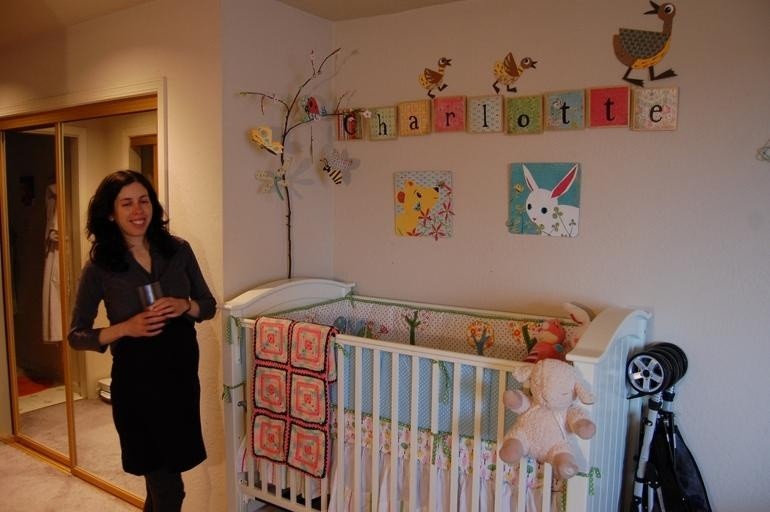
[184,296,191,313]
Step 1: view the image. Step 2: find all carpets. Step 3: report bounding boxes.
[15,369,60,397]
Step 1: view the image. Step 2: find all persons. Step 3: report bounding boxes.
[64,169,218,510]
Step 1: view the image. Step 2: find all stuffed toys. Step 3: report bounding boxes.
[564,302,593,347]
[500,357,597,479]
[525,318,566,365]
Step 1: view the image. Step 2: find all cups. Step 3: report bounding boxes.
[138,281,164,309]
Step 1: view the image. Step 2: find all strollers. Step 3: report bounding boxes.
[624,341,712,512]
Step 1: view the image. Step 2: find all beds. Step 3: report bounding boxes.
[223,277,650,512]
